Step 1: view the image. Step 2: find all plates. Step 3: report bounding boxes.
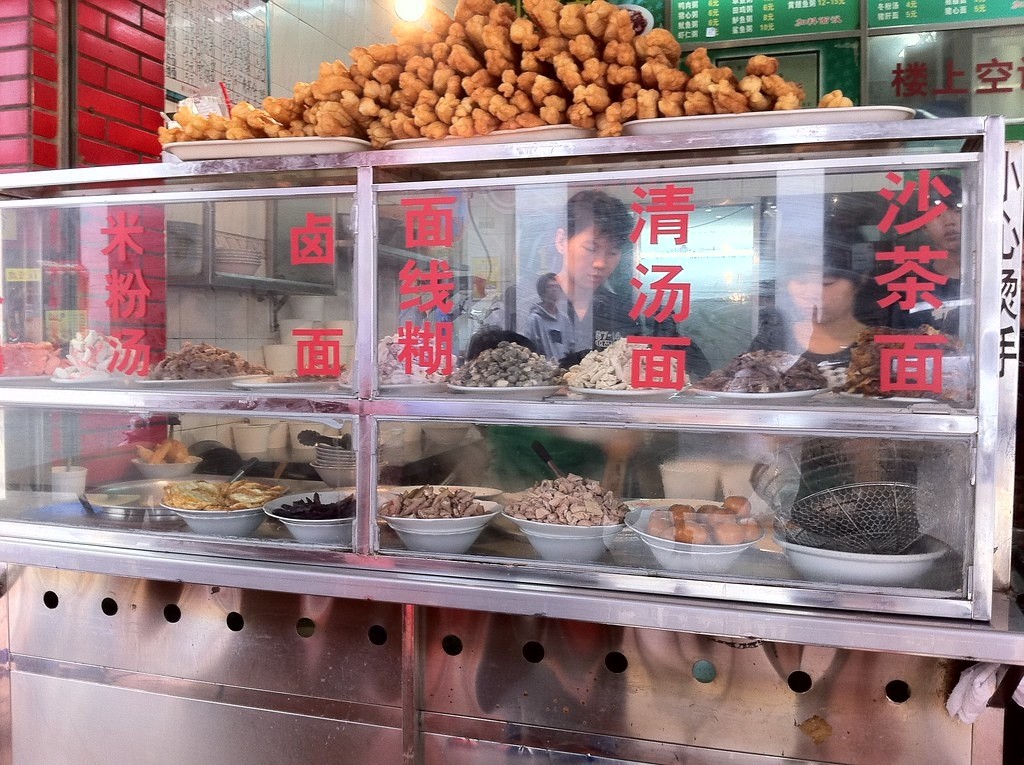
[616,4,654,37]
[3,372,938,406]
[390,485,503,497]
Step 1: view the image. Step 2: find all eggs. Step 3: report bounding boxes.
[647,496,763,546]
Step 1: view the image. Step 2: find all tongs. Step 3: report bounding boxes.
[296,430,352,450]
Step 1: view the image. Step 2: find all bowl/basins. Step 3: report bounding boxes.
[263,317,355,372]
[159,435,356,545]
[166,220,262,278]
[771,533,949,587]
[130,455,203,479]
[376,500,765,574]
[52,465,89,502]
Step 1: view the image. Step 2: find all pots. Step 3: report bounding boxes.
[80,480,219,529]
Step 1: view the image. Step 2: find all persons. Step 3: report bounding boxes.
[859,170,962,337]
[745,217,875,373]
[525,272,575,365]
[465,177,713,379]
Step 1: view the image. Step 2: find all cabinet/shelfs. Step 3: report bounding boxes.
[0,116,1004,622]
